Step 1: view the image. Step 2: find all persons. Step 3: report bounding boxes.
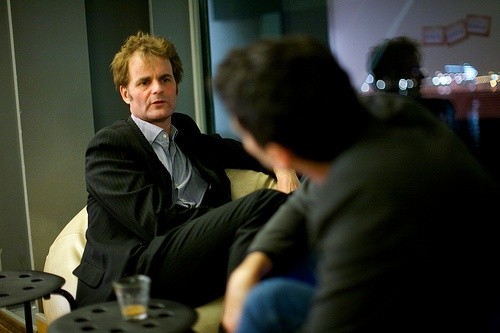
[369,39,427,99]
[212,31,500,333]
[73,32,306,311]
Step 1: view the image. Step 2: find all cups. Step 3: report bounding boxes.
[112,275,151,321]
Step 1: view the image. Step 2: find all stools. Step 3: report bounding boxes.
[46,298,198,332]
[0,269,76,332]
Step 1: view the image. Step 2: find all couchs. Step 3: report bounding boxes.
[35,168,278,333]
